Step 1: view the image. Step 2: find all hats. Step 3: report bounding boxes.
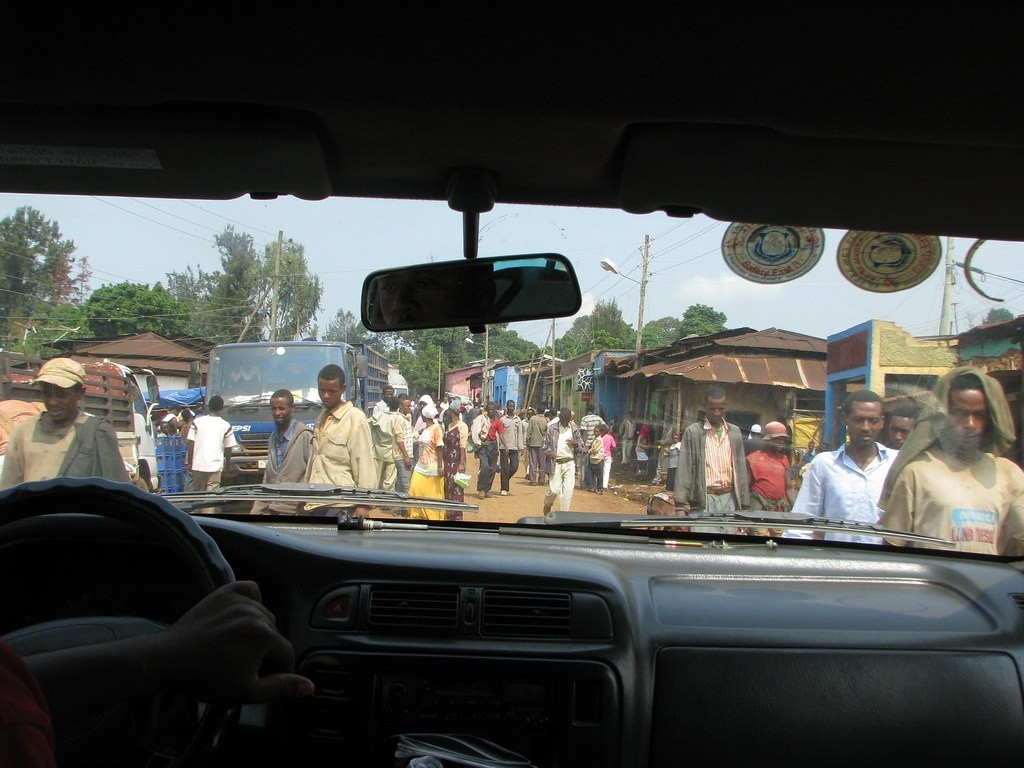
[35,357,88,391]
[449,400,465,414]
[751,424,762,433]
[763,421,790,441]
[419,395,436,406]
[422,404,439,419]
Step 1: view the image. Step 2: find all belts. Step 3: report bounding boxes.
[705,487,733,496]
[556,458,575,463]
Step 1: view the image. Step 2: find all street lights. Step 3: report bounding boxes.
[270,273,310,341]
[600,259,644,419]
[465,337,490,407]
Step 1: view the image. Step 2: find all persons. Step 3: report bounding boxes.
[803,441,828,462]
[376,267,453,326]
[609,412,657,476]
[294,364,377,519]
[0,581,315,768]
[0,357,131,489]
[367,385,468,521]
[666,435,681,491]
[877,365,1024,556]
[187,395,236,514]
[571,404,616,495]
[150,400,202,492]
[542,408,587,516]
[673,388,750,535]
[745,422,794,538]
[454,397,557,499]
[883,405,918,450]
[249,390,314,515]
[743,424,763,456]
[781,389,898,544]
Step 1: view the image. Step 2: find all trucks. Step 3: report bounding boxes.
[202,340,409,482]
[0,350,206,491]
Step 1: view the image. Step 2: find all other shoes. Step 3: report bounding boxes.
[485,491,492,498]
[530,482,536,486]
[539,482,545,486]
[543,506,551,517]
[500,490,508,496]
[479,491,485,499]
[525,474,530,480]
[596,489,603,495]
